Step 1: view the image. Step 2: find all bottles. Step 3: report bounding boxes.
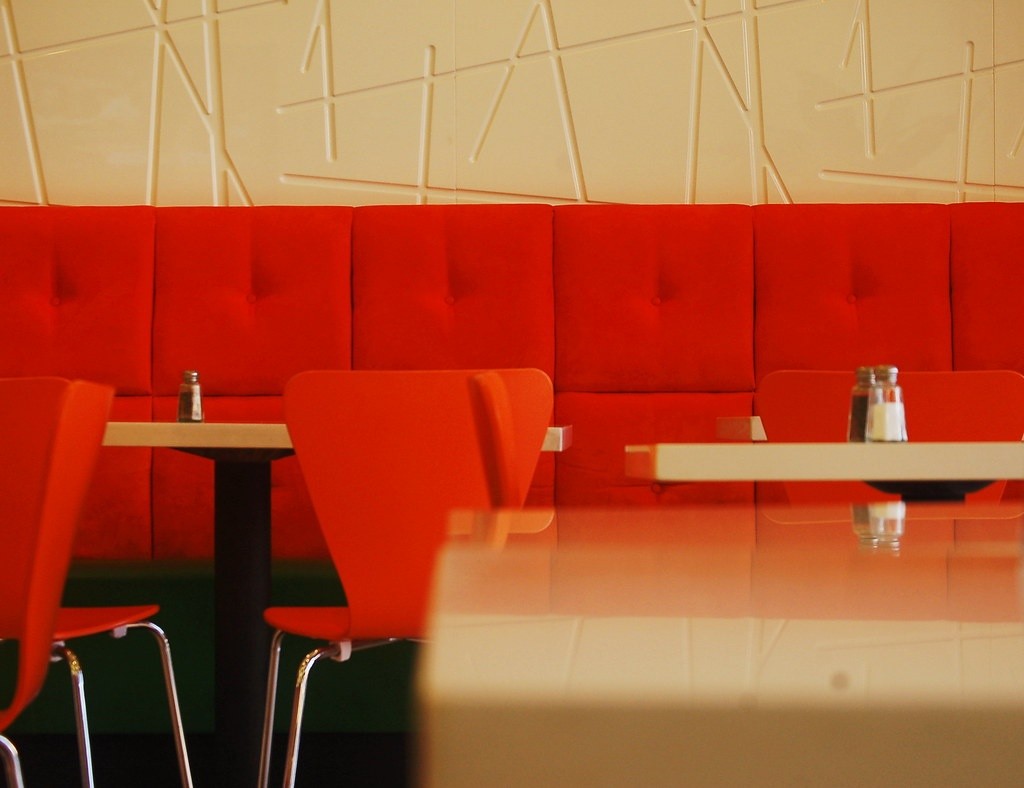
[866,365,908,441]
[178,371,203,423]
[852,506,877,550]
[848,368,873,442]
[869,502,906,558]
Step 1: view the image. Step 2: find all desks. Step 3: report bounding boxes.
[418,518,1024,788]
[102,421,575,788]
[623,442,1024,505]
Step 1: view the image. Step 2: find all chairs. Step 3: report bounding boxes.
[0,380,193,788]
[259,368,553,788]
[757,368,1024,511]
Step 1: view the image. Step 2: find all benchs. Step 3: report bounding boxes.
[0,202,1024,740]
